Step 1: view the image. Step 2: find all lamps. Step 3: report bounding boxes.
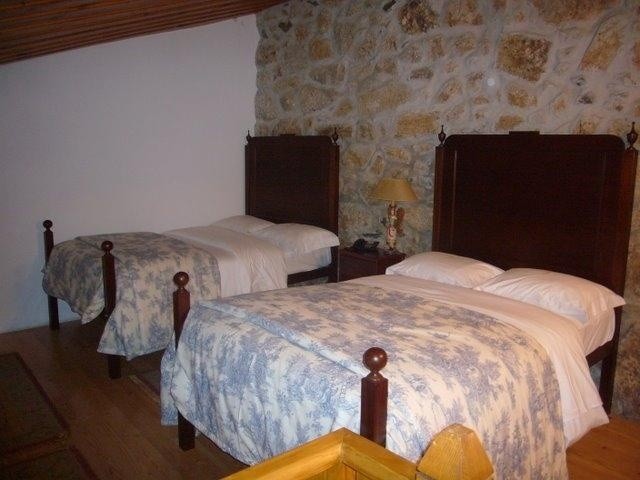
[368,177,417,256]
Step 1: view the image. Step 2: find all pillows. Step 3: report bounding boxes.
[386,250,628,328]
[211,215,340,255]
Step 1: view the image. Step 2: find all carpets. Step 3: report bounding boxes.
[1,352,100,480]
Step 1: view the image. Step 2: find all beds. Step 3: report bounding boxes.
[172,122,640,453]
[42,127,341,382]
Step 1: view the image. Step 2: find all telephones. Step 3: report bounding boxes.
[344,238,379,254]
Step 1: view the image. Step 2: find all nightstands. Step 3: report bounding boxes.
[339,247,406,283]
